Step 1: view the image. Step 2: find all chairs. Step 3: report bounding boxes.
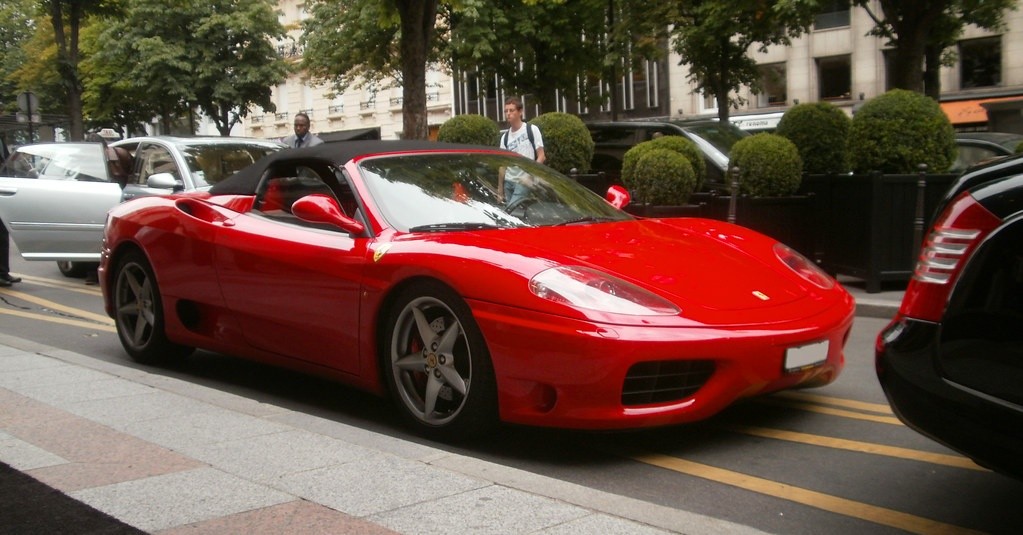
[263,178,359,219]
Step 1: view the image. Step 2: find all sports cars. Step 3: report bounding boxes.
[99,139,856,441]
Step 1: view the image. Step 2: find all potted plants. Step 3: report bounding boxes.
[437,89,961,294]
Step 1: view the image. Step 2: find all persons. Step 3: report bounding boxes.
[0,131,22,286]
[78,133,135,285]
[283,113,324,148]
[498,97,545,208]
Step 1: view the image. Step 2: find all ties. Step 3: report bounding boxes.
[297,139,303,147]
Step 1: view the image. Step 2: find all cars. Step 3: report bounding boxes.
[873,152,1023,484]
[28,155,72,179]
[576,120,754,196]
[1,137,290,278]
[951,133,1023,167]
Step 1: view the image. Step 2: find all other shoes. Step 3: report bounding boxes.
[0,274,22,286]
[86,272,96,285]
